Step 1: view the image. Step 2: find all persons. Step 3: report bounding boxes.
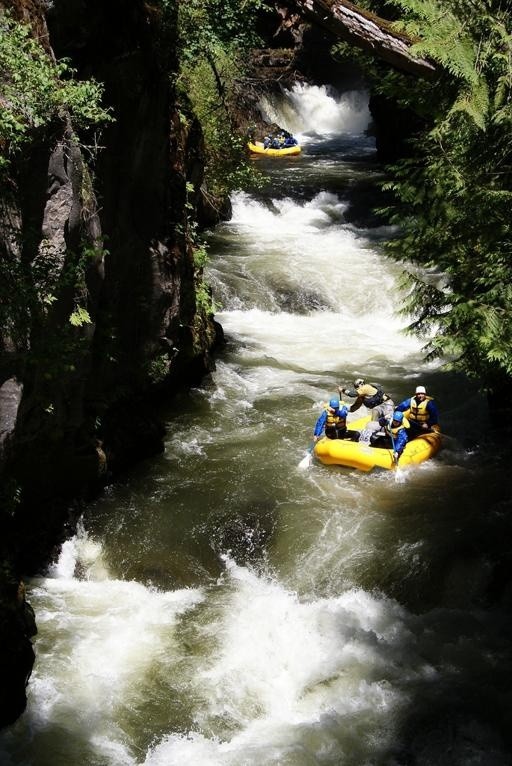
[394,386,438,441]
[370,411,407,462]
[313,397,360,442]
[262,129,298,148]
[337,377,395,422]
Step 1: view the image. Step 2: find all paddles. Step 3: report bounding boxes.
[298,443,315,468]
[386,419,405,484]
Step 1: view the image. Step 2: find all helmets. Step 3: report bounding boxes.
[393,411,403,422]
[416,386,426,394]
[330,399,339,408]
[354,379,365,386]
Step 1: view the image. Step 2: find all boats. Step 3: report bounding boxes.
[313,406,443,472]
[247,137,302,156]
